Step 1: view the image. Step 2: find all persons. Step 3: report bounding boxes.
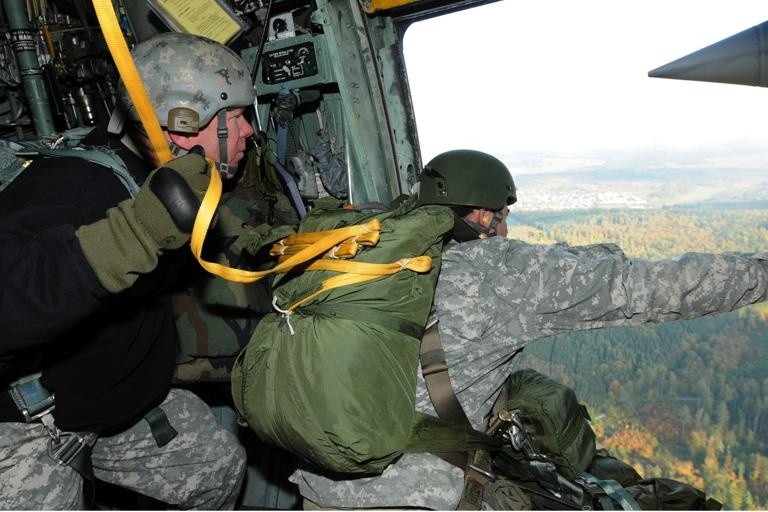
[0,34,259,510]
[287,149,766,510]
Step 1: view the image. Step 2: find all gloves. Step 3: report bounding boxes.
[78,146,221,295]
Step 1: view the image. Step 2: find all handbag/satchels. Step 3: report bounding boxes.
[506,369,596,473]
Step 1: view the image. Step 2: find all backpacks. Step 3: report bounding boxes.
[232,206,457,474]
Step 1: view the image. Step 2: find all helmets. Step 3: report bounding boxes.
[418,150,516,211]
[115,33,256,134]
